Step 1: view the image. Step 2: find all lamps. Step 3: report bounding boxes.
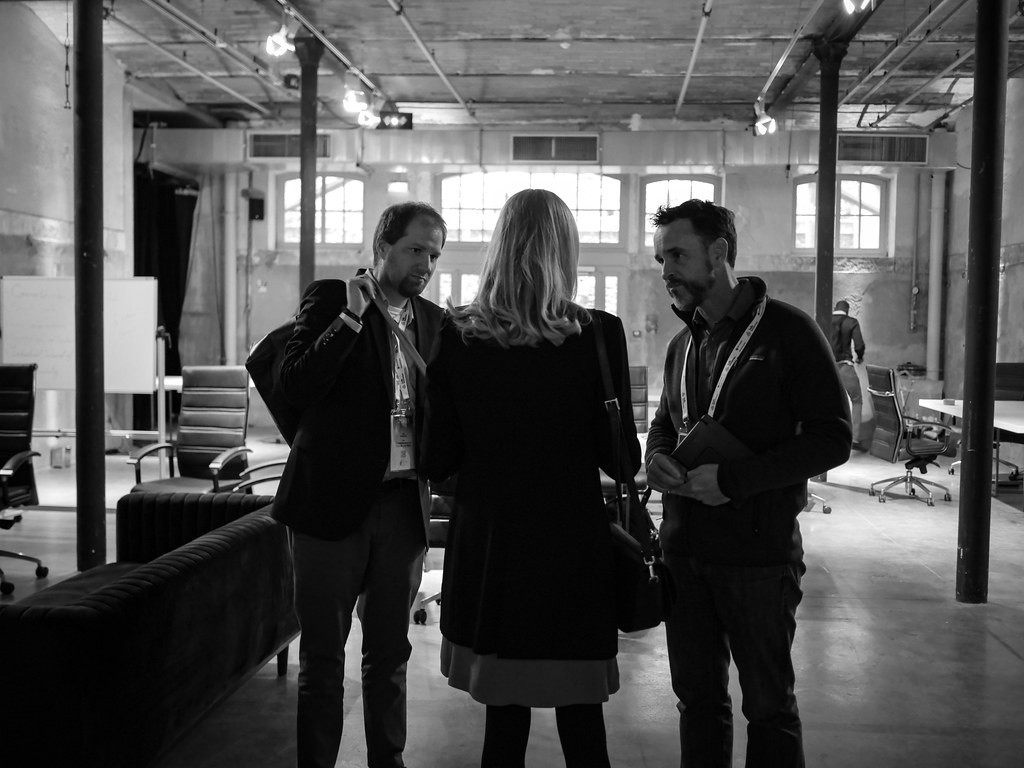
[377,110,412,130]
[265,5,288,56]
[756,95,776,135]
[844,0,876,15]
[358,89,386,129]
[344,69,368,113]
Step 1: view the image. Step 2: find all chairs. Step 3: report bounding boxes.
[865,363,1024,506]
[129,365,287,492]
[414,483,451,625]
[0,364,49,595]
[602,366,661,545]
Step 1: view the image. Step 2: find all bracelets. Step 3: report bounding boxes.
[342,308,365,325]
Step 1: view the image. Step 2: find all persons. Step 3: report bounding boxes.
[643,198,852,768]
[832,300,869,453]
[269,201,454,768]
[420,190,642,767]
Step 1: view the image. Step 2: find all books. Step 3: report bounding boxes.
[668,416,754,470]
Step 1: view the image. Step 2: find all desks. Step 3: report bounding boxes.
[148,375,255,457]
[918,399,1024,514]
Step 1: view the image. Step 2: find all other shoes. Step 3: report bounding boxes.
[852,440,866,452]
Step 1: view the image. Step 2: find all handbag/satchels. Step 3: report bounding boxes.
[606,493,662,558]
[246,322,300,446]
[610,521,675,633]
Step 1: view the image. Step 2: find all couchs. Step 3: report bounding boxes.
[0,491,301,768]
